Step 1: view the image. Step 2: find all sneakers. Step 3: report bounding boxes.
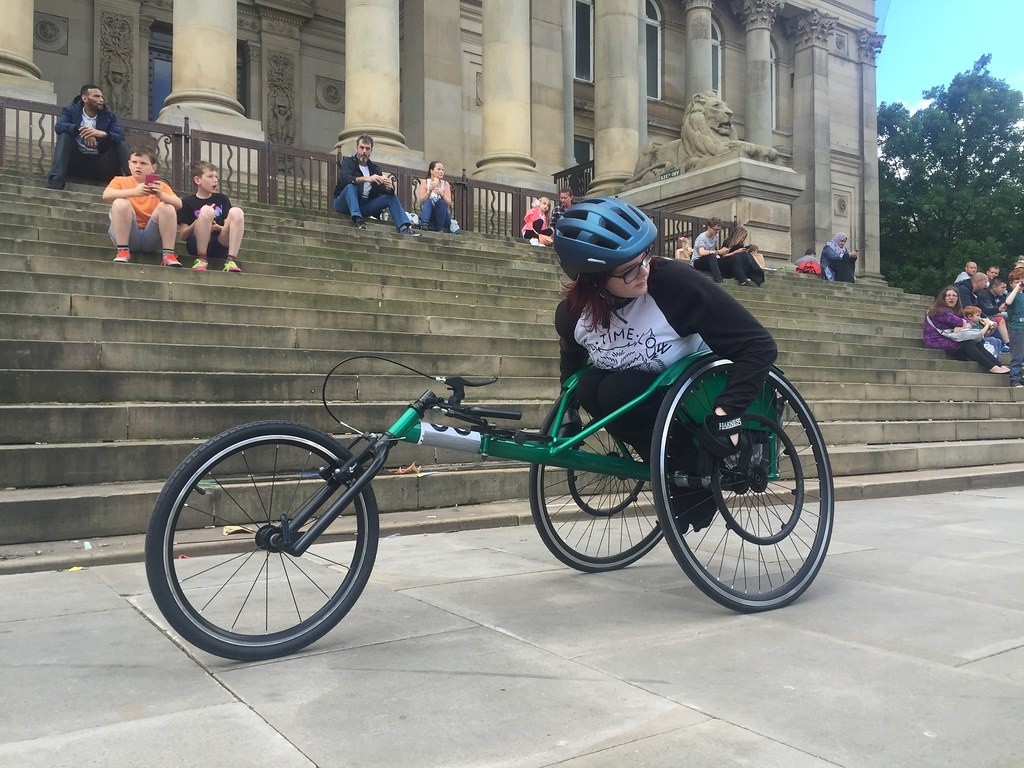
[222,261,241,272]
[160,253,183,267]
[113,250,131,262]
[192,258,209,270]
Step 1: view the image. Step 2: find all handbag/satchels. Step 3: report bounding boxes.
[796,261,821,276]
[538,233,553,245]
[942,325,984,342]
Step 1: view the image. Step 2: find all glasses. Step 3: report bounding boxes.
[945,294,957,298]
[600,241,657,284]
[710,227,721,231]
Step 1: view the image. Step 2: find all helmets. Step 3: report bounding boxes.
[553,197,658,281]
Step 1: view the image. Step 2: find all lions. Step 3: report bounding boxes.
[624,90,783,185]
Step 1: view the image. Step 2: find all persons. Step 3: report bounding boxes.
[820,232,858,283]
[794,249,820,265]
[675,237,694,262]
[690,216,756,287]
[550,187,574,234]
[722,226,768,288]
[102,144,182,267]
[333,135,422,237]
[923,255,1024,387]
[521,196,555,247]
[419,161,452,233]
[552,197,777,532]
[47,84,131,189]
[176,161,245,271]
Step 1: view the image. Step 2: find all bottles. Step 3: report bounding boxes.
[979,317,994,330]
[434,177,440,189]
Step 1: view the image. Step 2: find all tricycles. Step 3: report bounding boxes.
[144,349,840,663]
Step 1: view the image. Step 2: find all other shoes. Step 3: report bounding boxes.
[529,236,546,248]
[1001,342,1010,352]
[356,220,367,229]
[988,365,1006,374]
[1000,363,1011,372]
[401,227,422,237]
[741,281,750,286]
[1009,381,1024,388]
[50,174,63,189]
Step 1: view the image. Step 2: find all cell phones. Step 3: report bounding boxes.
[744,246,750,250]
[856,250,859,252]
[145,174,159,186]
